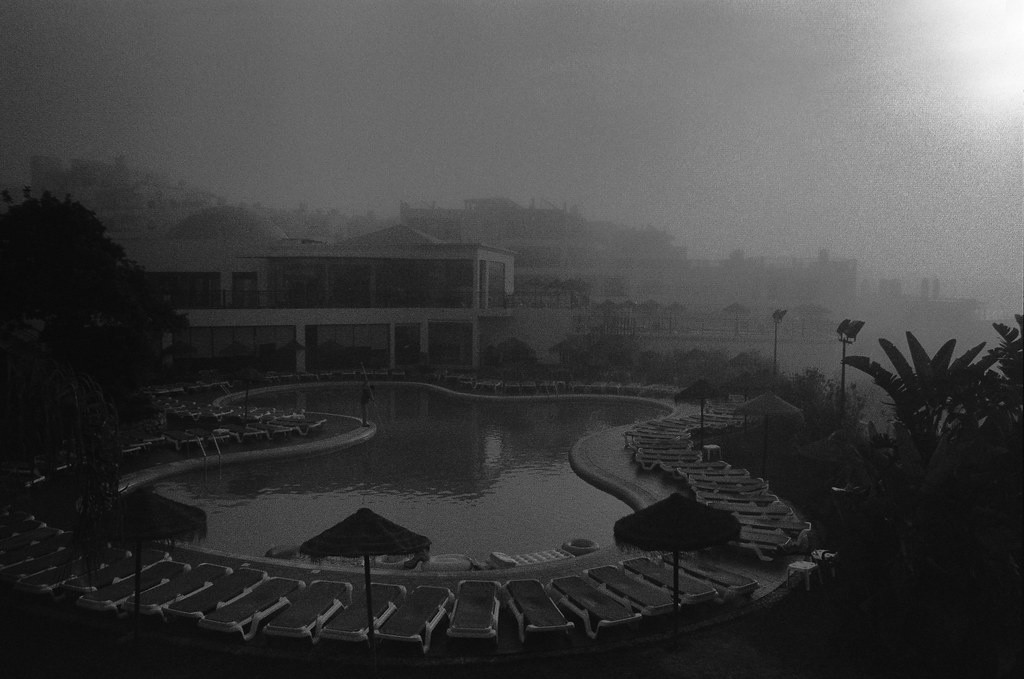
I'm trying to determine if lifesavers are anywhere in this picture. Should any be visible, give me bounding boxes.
[374,552,415,569]
[561,538,601,557]
[265,543,302,560]
[420,552,473,572]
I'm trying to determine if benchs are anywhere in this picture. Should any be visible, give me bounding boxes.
[620,381,796,516]
[545,574,643,641]
[446,579,504,648]
[656,552,762,607]
[78,558,192,620]
[501,578,575,645]
[582,564,682,623]
[261,579,354,646]
[328,367,622,396]
[121,562,235,622]
[161,567,269,623]
[374,584,456,655]
[197,576,307,641]
[0,438,170,606]
[729,511,813,547]
[320,582,407,649]
[117,368,328,460]
[618,556,719,615]
[727,526,792,563]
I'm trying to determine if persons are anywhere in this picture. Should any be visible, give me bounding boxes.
[360,379,376,426]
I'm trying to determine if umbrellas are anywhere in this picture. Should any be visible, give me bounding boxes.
[299,507,432,676]
[613,493,743,629]
[674,378,729,450]
[89,487,208,644]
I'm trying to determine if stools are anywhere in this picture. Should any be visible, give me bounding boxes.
[785,560,825,592]
